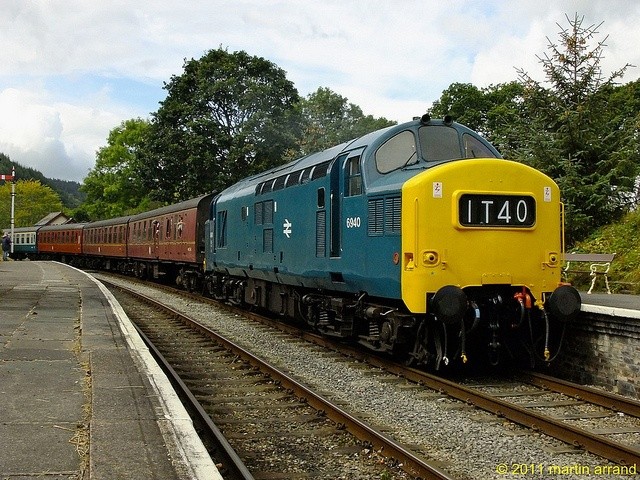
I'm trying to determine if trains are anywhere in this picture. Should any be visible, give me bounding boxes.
[0,114,582,381]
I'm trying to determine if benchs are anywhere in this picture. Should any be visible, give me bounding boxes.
[561,254,616,294]
